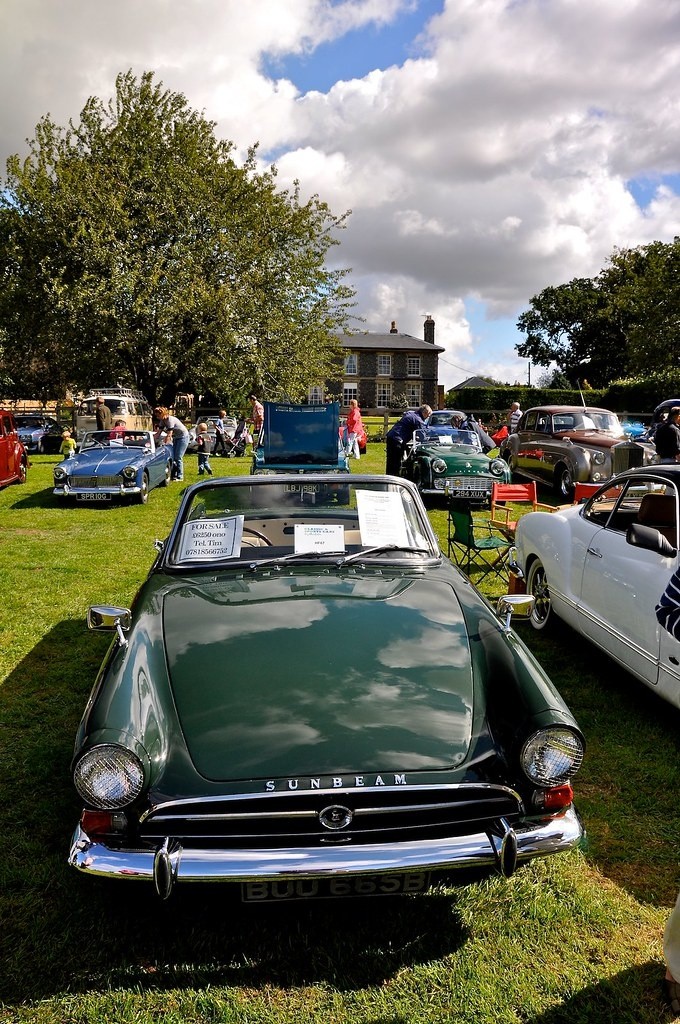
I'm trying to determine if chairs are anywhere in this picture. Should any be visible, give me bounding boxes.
[637,494,676,547]
[488,480,558,566]
[448,481,516,585]
[556,482,623,511]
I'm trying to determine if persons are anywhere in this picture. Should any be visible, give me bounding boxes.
[653,406,680,496]
[450,415,496,455]
[58,395,264,482]
[506,402,523,434]
[344,399,362,459]
[384,403,432,481]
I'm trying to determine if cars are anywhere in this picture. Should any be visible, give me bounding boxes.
[631,399,680,466]
[14,414,64,455]
[188,416,237,454]
[399,429,511,510]
[500,405,658,504]
[66,473,588,900]
[339,420,366,454]
[53,431,174,504]
[250,400,350,505]
[0,409,30,488]
[514,464,680,704]
[422,410,478,446]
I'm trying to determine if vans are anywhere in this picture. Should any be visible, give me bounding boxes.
[76,388,154,441]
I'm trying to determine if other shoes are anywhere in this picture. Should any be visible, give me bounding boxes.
[198,470,204,475]
[174,478,184,481]
[213,452,216,456]
[208,469,212,474]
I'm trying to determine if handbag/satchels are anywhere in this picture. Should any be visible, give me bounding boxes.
[245,434,253,444]
